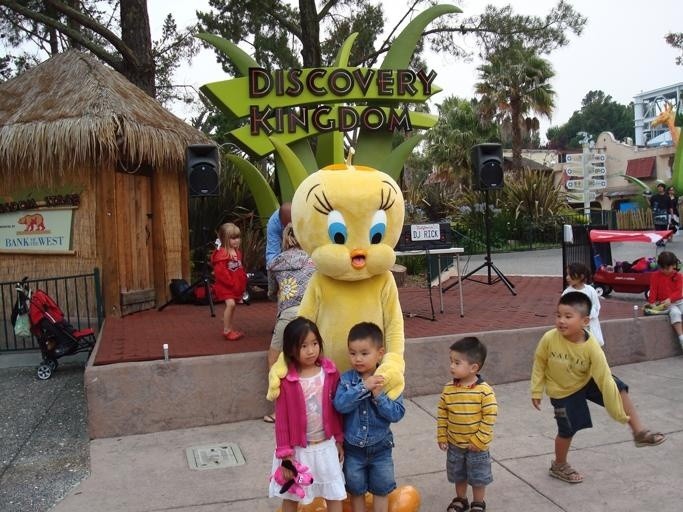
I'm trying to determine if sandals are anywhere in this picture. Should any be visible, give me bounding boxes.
[469,500,486,512]
[447,496,470,512]
[548,459,583,483]
[632,428,668,448]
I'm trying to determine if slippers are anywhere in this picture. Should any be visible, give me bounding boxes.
[263,415,276,424]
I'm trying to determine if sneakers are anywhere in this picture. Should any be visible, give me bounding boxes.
[222,329,243,340]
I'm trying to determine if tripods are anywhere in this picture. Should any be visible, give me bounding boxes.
[157,197,250,317]
[441,191,517,297]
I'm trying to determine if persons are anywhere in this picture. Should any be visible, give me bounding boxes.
[648,252,683,347]
[561,263,604,340]
[264,225,317,425]
[644,184,673,246]
[211,224,247,340]
[531,293,663,483]
[667,186,679,233]
[265,203,292,267]
[438,336,498,512]
[268,316,348,512]
[334,322,405,512]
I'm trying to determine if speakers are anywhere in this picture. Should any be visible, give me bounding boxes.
[470,142,505,192]
[184,145,222,197]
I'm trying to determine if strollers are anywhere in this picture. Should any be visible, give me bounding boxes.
[14,275,96,380]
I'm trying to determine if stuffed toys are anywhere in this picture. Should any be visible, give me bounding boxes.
[269,460,313,497]
[266,163,406,400]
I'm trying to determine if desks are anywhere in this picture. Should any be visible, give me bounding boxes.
[394,248,465,318]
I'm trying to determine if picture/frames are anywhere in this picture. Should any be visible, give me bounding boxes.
[0,183,83,256]
